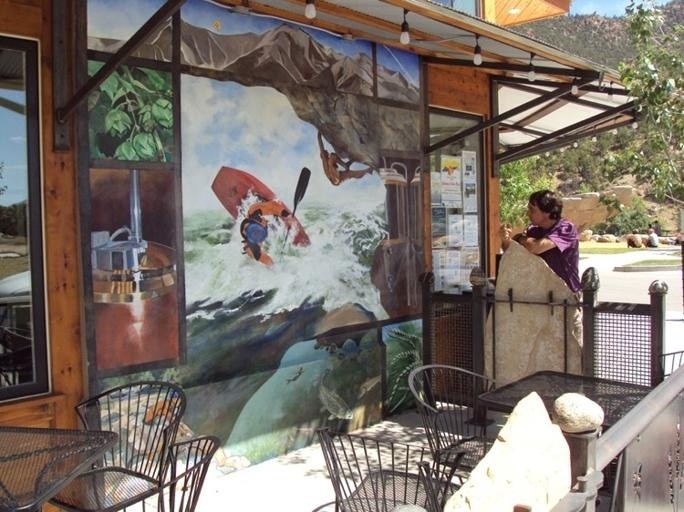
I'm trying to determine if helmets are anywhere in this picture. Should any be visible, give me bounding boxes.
[246,225,265,243]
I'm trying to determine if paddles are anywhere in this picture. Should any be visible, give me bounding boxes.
[283,167,312,245]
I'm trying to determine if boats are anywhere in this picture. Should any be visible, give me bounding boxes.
[210,165,310,247]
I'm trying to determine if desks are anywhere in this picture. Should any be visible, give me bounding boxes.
[477,370,656,432]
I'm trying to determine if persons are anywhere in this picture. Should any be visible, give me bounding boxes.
[499,184,581,309]
[234,199,298,266]
[626,220,683,248]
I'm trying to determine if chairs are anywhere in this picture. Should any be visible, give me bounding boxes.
[312,362,505,511]
[0,378,220,511]
